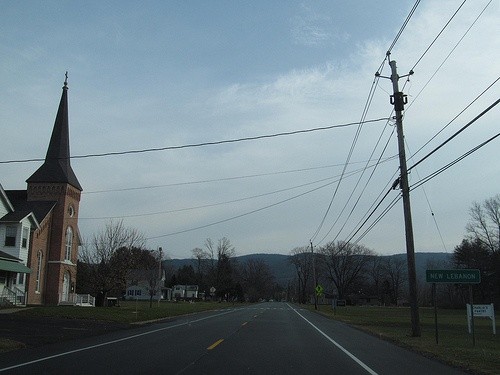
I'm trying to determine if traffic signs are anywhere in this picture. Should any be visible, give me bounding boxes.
[425,269,481,284]
